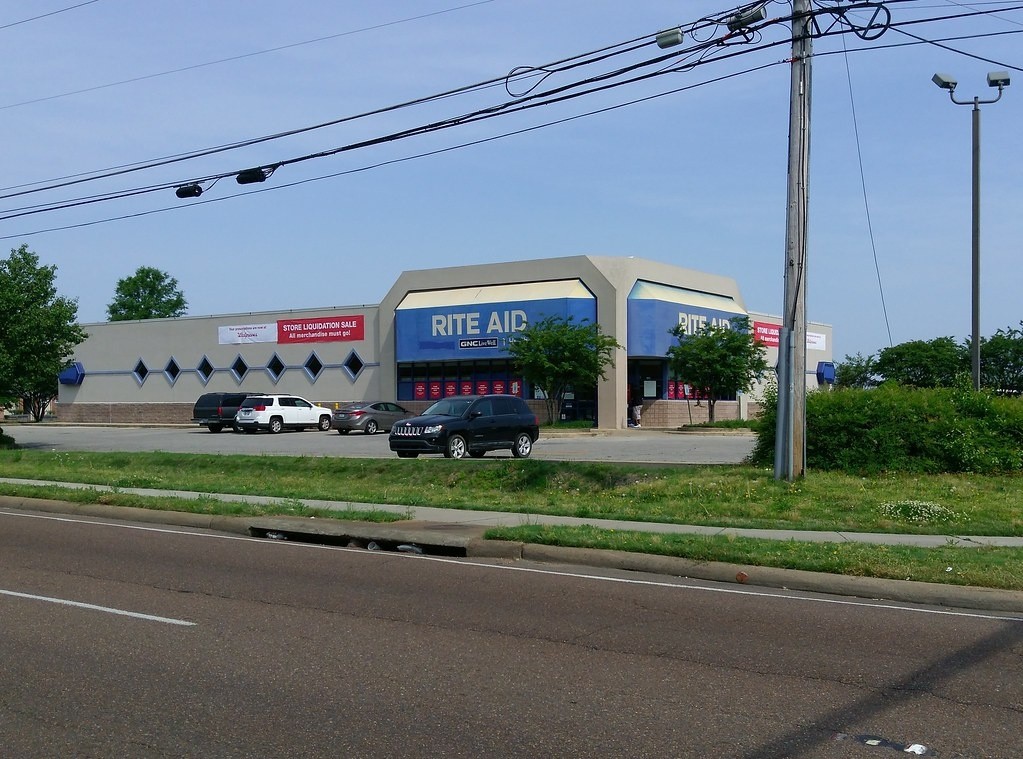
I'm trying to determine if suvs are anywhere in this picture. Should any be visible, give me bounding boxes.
[235,393,332,434]
[388,394,540,459]
[190,392,258,433]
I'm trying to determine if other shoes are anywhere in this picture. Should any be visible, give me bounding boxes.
[634,424,641,428]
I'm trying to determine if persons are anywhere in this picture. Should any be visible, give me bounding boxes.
[631,383,644,427]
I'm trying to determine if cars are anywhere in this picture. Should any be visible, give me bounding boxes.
[332,400,415,435]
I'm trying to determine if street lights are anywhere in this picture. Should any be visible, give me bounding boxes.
[931,71,1011,394]
